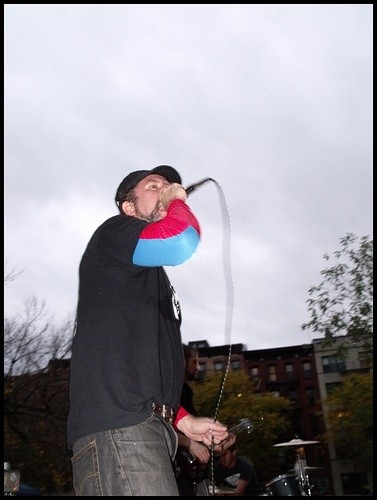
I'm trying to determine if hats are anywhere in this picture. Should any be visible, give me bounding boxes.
[117,165,182,207]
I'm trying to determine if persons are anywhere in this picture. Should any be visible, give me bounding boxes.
[66,164,228,496]
[218,444,261,496]
[175,344,199,495]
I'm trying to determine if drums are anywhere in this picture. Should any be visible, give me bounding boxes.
[264,473,307,497]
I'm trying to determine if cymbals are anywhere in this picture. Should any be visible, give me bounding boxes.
[290,466,323,472]
[273,438,321,446]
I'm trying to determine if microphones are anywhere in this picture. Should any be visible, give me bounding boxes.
[158,184,196,211]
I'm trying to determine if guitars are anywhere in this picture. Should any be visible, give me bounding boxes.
[176,415,253,482]
